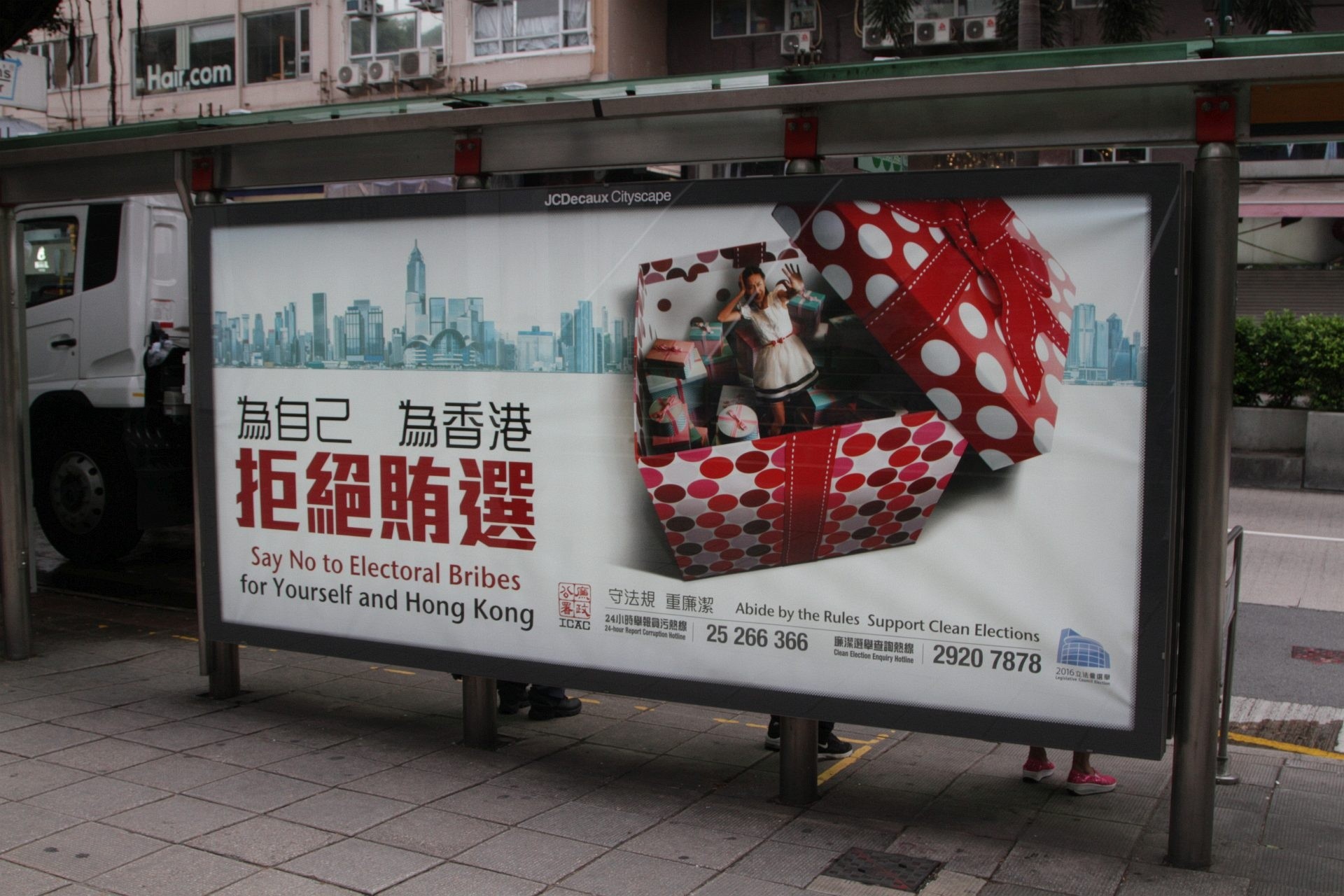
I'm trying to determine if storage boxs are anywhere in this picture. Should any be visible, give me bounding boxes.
[632,198,1076,582]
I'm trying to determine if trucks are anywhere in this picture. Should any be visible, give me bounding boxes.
[15,194,238,570]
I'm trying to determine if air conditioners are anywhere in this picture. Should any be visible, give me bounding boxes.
[914,18,949,45]
[367,59,394,83]
[337,64,363,87]
[780,31,812,55]
[964,17,1002,41]
[399,47,437,79]
[862,25,900,48]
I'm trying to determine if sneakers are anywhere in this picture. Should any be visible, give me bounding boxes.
[1066,767,1117,795]
[817,732,852,760]
[765,722,780,750]
[1022,756,1054,785]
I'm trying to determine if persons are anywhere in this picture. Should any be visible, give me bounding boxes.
[764,713,854,760]
[1022,742,1117,795]
[496,679,583,721]
[717,263,821,437]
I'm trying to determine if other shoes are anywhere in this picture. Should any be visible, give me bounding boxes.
[528,697,582,722]
[497,690,531,715]
